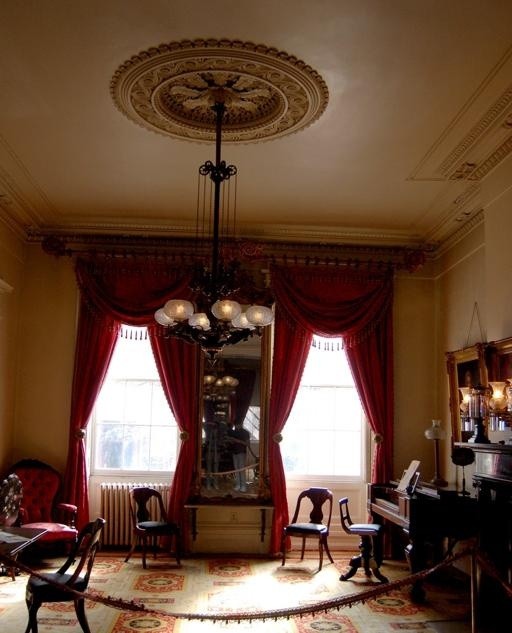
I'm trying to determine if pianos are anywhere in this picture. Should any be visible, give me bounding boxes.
[366,469,458,602]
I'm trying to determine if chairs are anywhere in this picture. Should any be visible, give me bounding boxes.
[283,486,389,587]
[123,487,182,569]
[0,457,105,633]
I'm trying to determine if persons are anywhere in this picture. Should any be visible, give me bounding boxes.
[205,420,223,489]
[225,415,250,491]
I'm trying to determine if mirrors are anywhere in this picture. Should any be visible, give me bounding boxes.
[200,325,267,503]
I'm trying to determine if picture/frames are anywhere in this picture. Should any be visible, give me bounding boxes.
[447,340,512,444]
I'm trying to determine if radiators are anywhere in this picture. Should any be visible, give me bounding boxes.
[100,483,174,550]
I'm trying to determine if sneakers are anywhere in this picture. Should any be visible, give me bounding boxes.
[233,485,247,492]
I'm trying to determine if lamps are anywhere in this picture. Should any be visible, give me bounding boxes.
[204,350,238,405]
[426,418,448,485]
[458,387,472,420]
[154,103,276,366]
[488,380,512,426]
